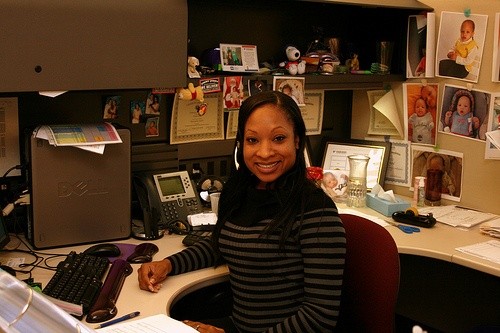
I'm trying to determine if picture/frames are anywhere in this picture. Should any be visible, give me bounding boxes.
[321,141,392,194]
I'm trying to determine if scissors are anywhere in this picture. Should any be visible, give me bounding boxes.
[384,220,420,234]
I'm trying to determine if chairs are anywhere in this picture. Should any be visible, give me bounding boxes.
[203,214,401,333]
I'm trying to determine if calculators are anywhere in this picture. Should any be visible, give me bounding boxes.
[182,224,216,247]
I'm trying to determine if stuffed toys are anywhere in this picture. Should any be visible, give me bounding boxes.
[280,46,307,76]
[188,57,199,73]
[177,84,204,103]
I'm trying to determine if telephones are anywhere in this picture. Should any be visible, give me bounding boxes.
[134,168,203,227]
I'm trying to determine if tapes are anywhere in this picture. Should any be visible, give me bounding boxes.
[405,208,419,216]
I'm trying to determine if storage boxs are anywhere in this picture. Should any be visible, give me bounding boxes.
[0,96,21,178]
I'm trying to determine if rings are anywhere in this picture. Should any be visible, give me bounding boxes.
[196,325,200,330]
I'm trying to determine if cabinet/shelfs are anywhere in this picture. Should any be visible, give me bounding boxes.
[0,0,435,93]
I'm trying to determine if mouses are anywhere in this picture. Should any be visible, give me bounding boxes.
[83,244,121,257]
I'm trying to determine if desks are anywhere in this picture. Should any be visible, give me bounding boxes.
[0,189,500,333]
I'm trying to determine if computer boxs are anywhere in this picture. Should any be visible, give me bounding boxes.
[25,123,133,250]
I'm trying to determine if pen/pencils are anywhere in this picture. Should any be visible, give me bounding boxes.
[94,311,140,329]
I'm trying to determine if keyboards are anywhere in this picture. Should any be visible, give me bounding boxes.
[42,251,110,314]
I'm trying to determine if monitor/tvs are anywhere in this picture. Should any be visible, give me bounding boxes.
[0,206,10,249]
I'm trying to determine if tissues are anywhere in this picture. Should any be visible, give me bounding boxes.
[365,182,411,217]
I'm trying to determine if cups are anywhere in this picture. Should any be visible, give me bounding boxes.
[424,169,444,205]
[210,192,221,212]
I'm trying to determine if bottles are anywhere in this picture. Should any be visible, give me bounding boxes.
[348,155,370,207]
[413,176,425,208]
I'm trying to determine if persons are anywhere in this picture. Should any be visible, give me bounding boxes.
[138,90,346,333]
[409,97,434,146]
[225,79,240,109]
[444,90,480,138]
[283,85,299,105]
[429,156,455,196]
[322,172,349,198]
[227,50,235,65]
[109,100,118,119]
[437,20,478,79]
[421,84,437,108]
[104,99,112,118]
